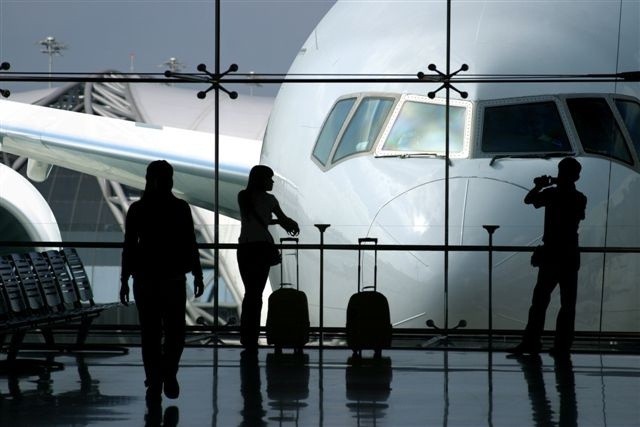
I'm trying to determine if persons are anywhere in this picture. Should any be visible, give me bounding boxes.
[236,164,300,350]
[508,157,588,355]
[120,160,205,410]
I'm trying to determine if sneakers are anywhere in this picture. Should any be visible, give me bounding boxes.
[550,347,570,361]
[162,363,179,398]
[145,388,161,406]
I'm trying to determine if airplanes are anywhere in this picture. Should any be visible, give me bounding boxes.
[0,1,640,332]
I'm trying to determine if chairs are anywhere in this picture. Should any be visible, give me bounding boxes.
[0,247,128,357]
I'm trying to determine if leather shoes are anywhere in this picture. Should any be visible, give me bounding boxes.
[506,341,543,355]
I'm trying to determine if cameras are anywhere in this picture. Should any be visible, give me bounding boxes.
[534,175,558,186]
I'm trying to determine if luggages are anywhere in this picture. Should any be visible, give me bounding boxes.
[266,238,309,354]
[346,238,392,360]
